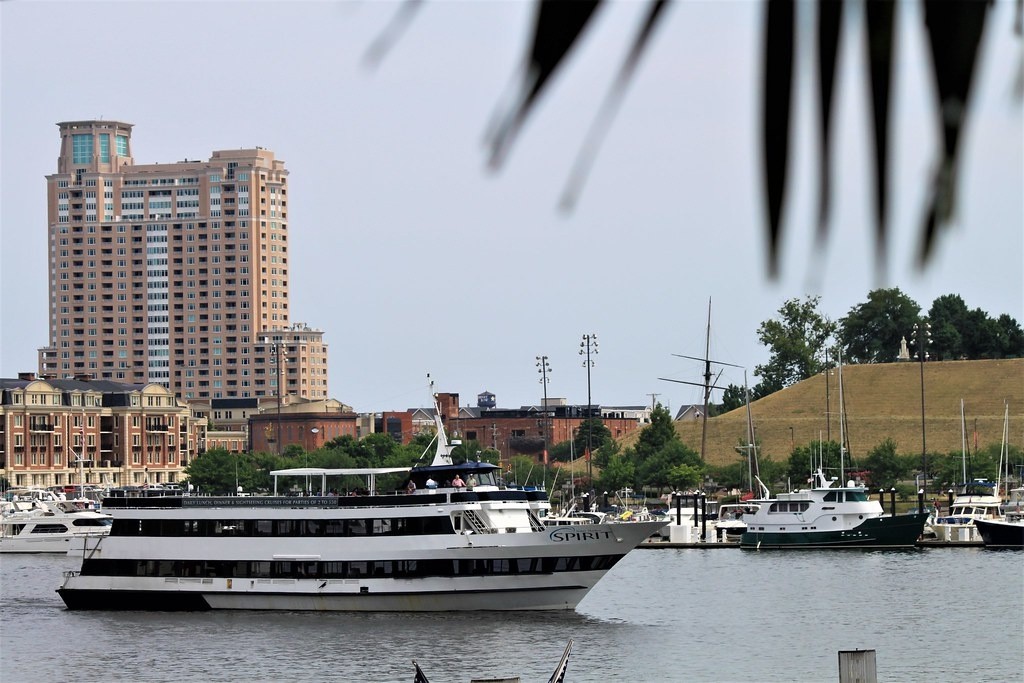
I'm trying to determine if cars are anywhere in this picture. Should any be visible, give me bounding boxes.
[22,484,183,501]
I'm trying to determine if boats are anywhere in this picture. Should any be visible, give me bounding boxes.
[58,373,674,611]
[0,498,113,554]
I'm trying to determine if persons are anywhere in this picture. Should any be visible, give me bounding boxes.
[425,475,438,489]
[303,487,370,496]
[452,474,466,487]
[466,474,476,486]
[407,480,416,493]
[932,498,941,512]
[13,494,18,500]
[84,497,89,509]
[695,411,699,418]
[734,508,744,514]
[444,480,452,487]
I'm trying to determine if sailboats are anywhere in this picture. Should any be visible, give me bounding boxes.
[740,348,1024,549]
